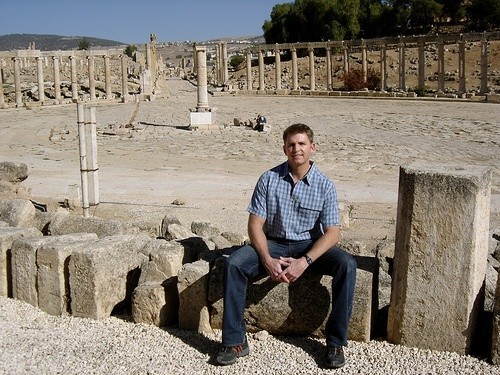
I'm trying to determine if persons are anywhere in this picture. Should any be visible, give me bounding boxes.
[214,123,357,369]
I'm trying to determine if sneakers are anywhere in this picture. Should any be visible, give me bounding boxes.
[325,343,344,368]
[215,335,249,365]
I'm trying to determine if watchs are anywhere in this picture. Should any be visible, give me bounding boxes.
[302,254,313,265]
